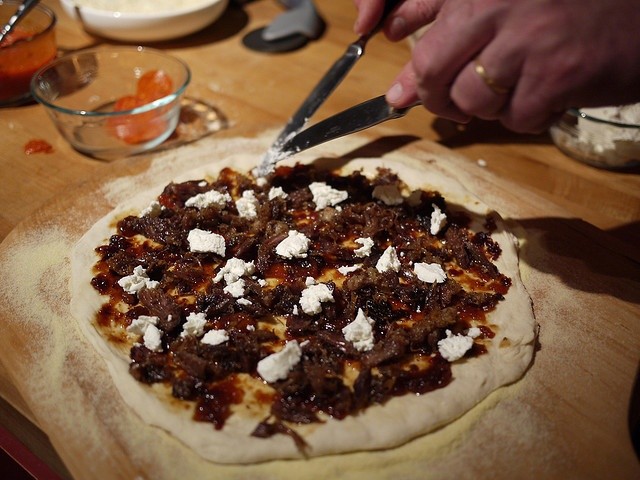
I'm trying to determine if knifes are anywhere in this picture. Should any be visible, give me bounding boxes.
[257,16,384,176]
[273,90,423,163]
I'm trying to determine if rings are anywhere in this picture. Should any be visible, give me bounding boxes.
[474,57,509,97]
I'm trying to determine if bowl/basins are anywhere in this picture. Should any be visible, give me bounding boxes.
[59,1,225,44]
[549,103,639,170]
[31,45,191,162]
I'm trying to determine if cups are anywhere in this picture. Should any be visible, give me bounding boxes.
[0,1,56,104]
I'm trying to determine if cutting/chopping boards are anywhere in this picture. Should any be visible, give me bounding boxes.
[0,1,638,479]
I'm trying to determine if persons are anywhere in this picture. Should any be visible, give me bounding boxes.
[353,0,640,135]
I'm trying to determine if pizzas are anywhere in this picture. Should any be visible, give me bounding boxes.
[70,157,539,462]
